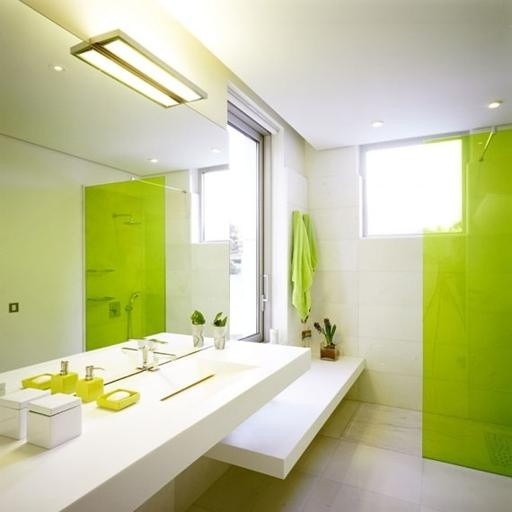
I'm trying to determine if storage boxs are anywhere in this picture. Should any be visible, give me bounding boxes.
[1,388,50,441]
[27,392,81,451]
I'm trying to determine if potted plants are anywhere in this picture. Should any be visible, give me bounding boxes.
[212,312,227,351]
[190,309,206,348]
[314,319,340,360]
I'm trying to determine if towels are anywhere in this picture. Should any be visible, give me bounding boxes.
[291,210,319,321]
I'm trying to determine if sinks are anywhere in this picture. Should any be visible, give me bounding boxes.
[143,362,241,407]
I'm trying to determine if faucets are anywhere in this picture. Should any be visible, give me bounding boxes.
[149,346,178,369]
[123,342,149,372]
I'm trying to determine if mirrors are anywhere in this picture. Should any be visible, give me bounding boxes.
[0,0,229,396]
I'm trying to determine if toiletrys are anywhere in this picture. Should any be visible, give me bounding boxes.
[51,360,79,395]
[76,365,106,402]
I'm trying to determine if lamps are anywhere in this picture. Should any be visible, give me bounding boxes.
[72,40,182,108]
[90,29,207,103]
